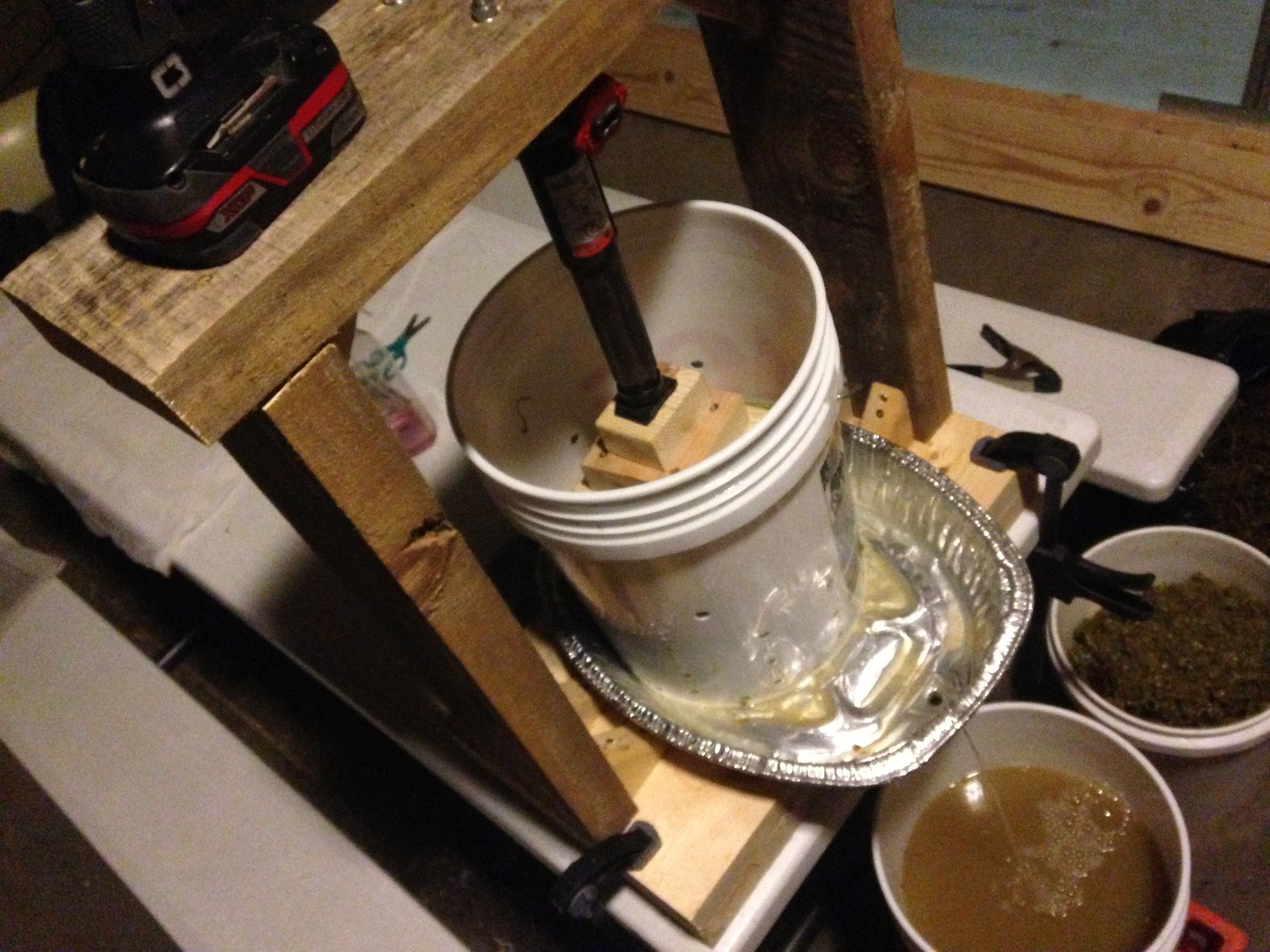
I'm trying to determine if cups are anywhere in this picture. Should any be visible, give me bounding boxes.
[348,329,437,455]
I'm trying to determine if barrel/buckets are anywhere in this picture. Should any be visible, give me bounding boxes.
[1045,526,1270,850]
[445,199,860,705]
[870,700,1191,952]
